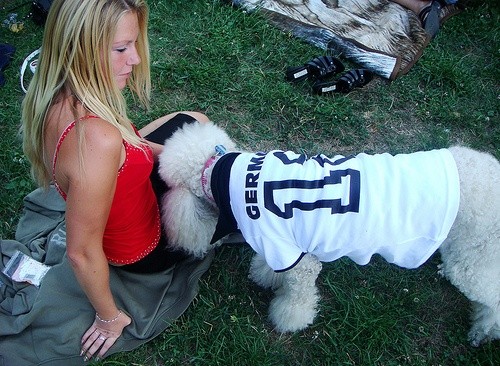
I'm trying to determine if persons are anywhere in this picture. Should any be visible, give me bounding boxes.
[393,0,441,38]
[21,0,210,363]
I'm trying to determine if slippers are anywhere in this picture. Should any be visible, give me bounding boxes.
[418,2,440,37]
[286,56,373,96]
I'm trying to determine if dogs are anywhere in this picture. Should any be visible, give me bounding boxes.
[158,120,500,348]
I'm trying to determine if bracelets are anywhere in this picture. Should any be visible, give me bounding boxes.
[96,311,122,323]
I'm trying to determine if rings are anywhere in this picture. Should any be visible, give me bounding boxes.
[98,337,106,342]
